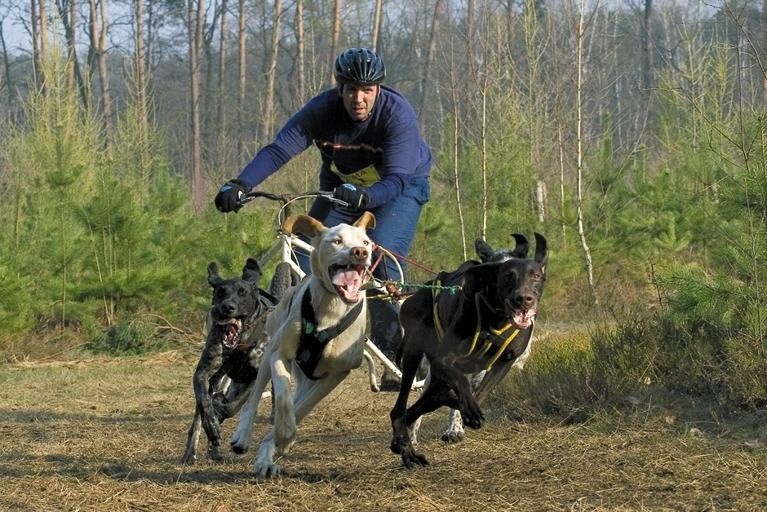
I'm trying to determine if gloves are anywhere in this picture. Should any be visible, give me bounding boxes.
[333,183,369,213]
[214,178,250,213]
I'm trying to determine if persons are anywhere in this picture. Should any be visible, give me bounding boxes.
[214,48,431,384]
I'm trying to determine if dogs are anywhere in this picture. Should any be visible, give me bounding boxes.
[180,256,279,466]
[230,210,381,479]
[389,232,550,471]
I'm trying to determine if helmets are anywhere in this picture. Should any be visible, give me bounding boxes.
[333,47,385,87]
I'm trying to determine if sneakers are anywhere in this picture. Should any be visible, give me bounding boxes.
[380,357,401,386]
